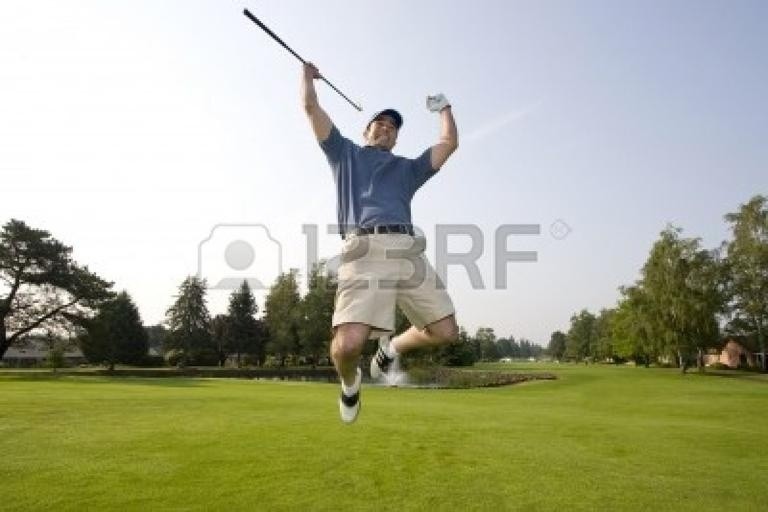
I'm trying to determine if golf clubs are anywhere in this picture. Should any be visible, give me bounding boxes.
[241,7,364,113]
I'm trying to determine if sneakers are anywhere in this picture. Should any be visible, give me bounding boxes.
[369,334,399,379]
[339,368,363,424]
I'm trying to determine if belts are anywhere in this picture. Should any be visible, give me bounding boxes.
[356,222,415,236]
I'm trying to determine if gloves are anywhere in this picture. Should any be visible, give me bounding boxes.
[425,92,451,113]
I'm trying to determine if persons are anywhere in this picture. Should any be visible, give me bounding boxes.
[301,61,458,424]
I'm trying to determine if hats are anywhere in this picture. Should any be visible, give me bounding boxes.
[366,108,402,129]
